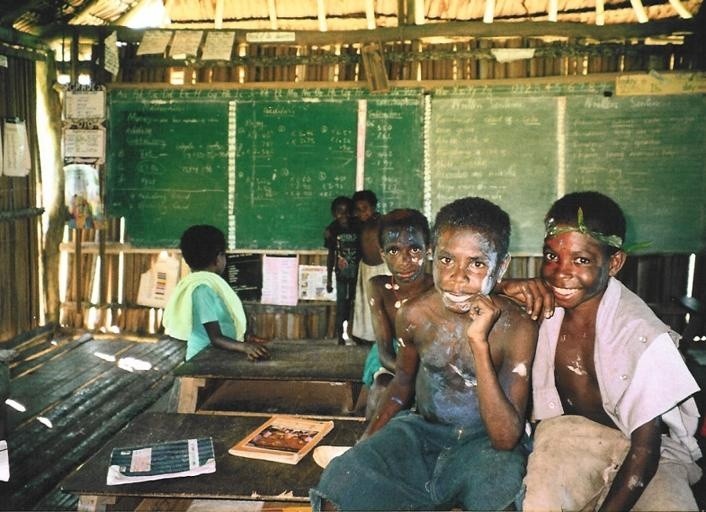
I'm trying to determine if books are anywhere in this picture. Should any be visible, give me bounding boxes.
[228,415,335,465]
[107,436,216,487]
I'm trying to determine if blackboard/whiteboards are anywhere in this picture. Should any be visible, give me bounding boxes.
[101,75,706,260]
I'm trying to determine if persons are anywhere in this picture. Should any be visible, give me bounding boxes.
[326,191,381,346]
[362,209,434,423]
[351,191,393,342]
[492,193,703,512]
[166,225,272,413]
[309,197,538,511]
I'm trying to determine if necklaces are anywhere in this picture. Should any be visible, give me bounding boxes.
[391,275,404,305]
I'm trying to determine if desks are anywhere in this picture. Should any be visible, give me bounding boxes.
[174,343,372,418]
[60,414,370,512]
